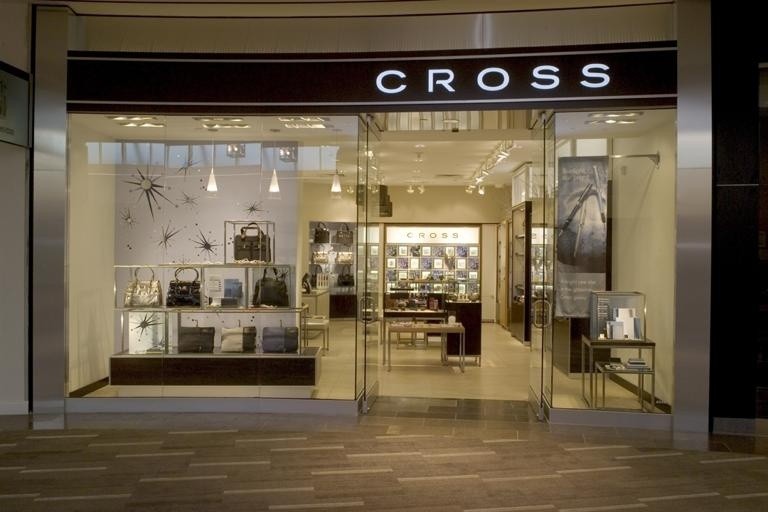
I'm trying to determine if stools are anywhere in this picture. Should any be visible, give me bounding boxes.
[298,316,330,357]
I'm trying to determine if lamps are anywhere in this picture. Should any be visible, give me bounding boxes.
[206,127,343,195]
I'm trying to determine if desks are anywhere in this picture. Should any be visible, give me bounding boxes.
[385,320,467,372]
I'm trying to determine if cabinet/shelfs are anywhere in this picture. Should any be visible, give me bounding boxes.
[113,264,308,352]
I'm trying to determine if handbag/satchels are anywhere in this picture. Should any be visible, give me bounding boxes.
[251,266,289,307]
[309,244,329,265]
[166,267,200,308]
[122,264,164,307]
[334,246,354,265]
[220,325,257,353]
[233,222,272,263]
[209,278,243,307]
[557,168,607,272]
[337,265,355,288]
[177,326,215,353]
[314,222,329,243]
[261,326,298,353]
[336,223,354,248]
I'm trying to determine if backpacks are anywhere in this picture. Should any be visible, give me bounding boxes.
[310,264,329,289]
